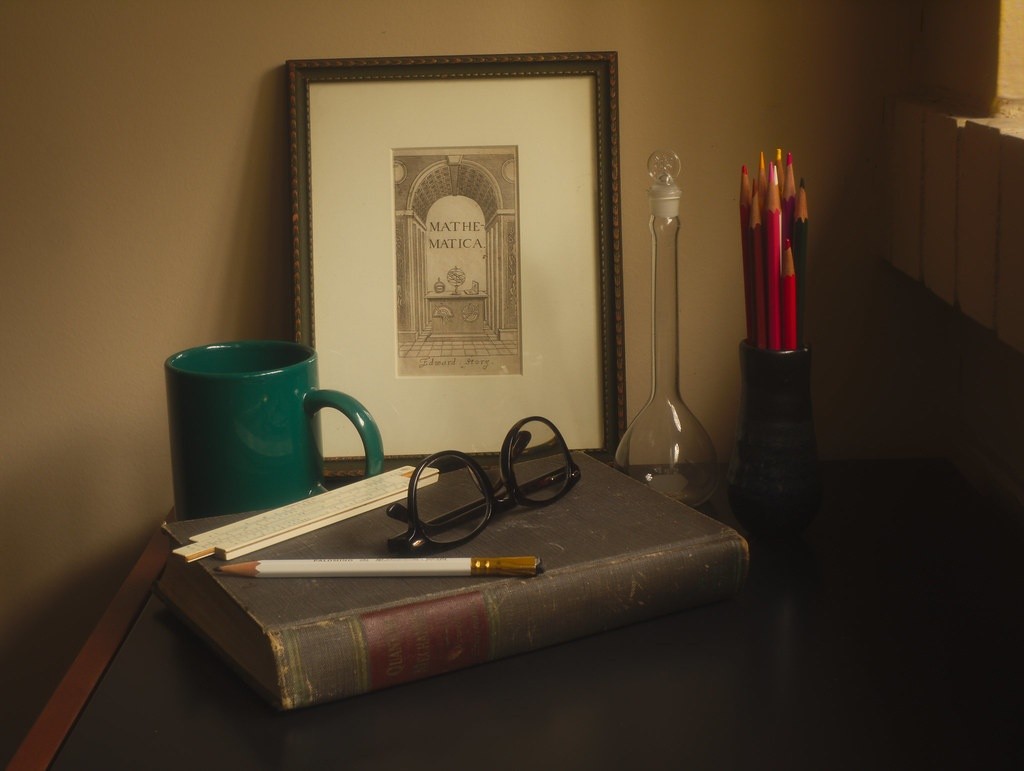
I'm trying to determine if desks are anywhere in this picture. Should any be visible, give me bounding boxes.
[0,454,1024,771]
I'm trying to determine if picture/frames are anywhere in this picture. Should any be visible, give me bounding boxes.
[286,52,629,476]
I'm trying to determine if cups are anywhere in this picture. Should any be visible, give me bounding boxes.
[163,339,384,522]
[729,335,822,546]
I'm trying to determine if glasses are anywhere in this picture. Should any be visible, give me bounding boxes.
[383,417,581,553]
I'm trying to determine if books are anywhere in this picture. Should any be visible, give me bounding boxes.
[158,452,751,711]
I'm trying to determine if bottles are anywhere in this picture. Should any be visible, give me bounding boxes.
[614,147,721,512]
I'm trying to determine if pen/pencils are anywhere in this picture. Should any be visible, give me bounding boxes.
[214,556,548,586]
[739,147,809,350]
